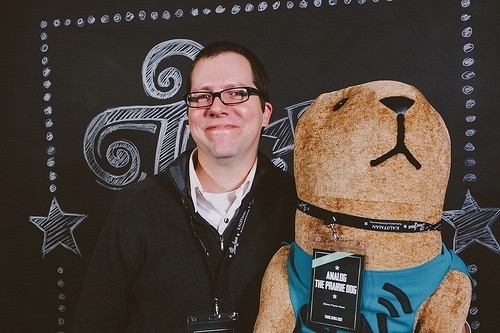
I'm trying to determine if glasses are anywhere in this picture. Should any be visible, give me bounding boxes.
[184,87,262,108]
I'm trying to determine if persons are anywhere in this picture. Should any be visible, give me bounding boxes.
[71,43,299,332]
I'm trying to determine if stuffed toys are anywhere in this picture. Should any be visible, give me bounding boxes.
[252,80,473,333]
